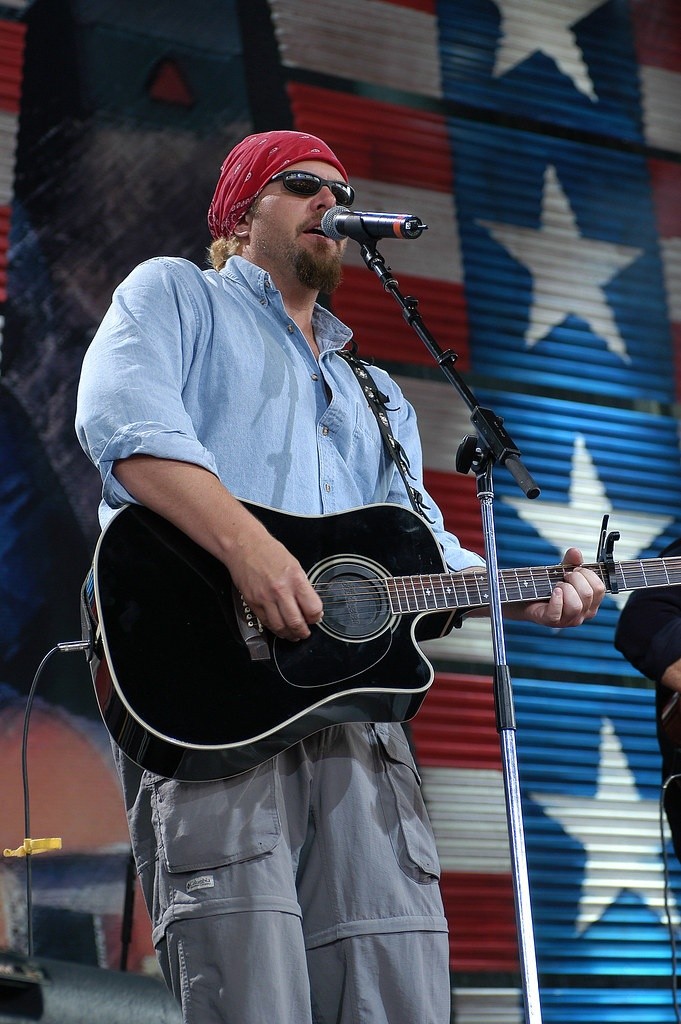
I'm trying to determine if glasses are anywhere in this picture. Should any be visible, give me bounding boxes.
[271,169,356,207]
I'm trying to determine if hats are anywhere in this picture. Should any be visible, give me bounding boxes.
[207,130,345,240]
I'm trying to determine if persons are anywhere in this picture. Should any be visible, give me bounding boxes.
[613,535,681,864]
[75,129,606,1024]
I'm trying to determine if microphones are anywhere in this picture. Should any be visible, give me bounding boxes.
[321,206,429,239]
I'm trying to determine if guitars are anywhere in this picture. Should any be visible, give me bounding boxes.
[80,487,681,790]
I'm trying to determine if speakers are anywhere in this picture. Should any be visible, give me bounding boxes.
[0,950,184,1024]
